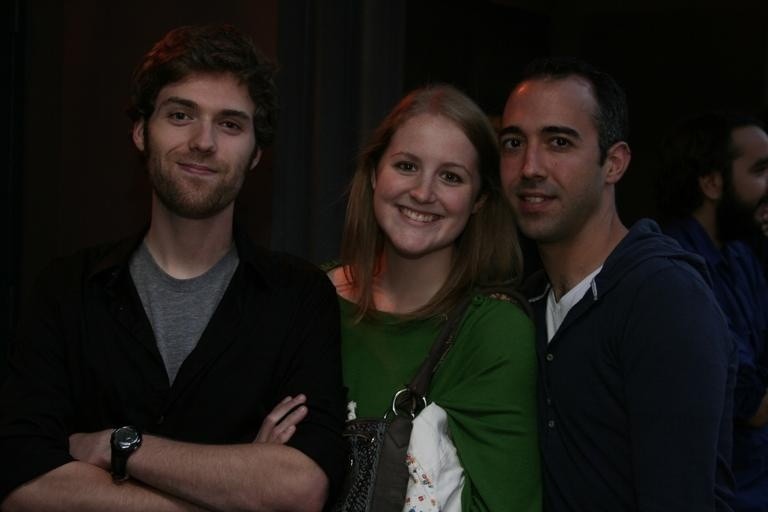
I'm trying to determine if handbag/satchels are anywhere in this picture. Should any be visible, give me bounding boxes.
[350,293,493,511]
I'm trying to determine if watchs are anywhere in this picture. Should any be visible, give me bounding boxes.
[109,425,146,481]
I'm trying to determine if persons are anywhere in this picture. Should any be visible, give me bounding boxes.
[0,24,350,512]
[318,84,543,512]
[660,120,768,512]
[494,61,740,512]
